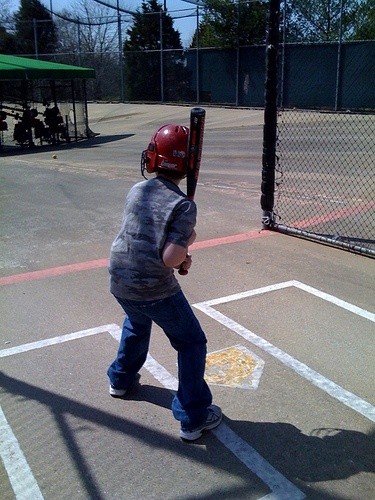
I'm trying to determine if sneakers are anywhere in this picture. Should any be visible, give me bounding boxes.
[109,384,127,396]
[179,404,223,440]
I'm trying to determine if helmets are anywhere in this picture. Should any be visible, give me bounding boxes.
[142,124,190,180]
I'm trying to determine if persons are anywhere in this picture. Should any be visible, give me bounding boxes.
[106,122,221,442]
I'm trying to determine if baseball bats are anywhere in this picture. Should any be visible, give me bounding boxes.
[178,108,206,275]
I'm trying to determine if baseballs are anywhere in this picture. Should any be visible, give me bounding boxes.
[52,154,57,159]
